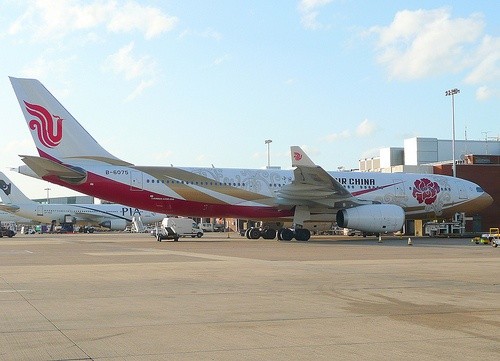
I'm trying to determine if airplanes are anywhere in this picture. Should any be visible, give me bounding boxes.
[0,171,166,233]
[5,74,493,242]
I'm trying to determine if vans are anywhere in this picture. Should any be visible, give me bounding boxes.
[161,216,203,238]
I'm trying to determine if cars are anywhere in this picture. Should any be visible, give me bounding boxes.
[490,236,500,247]
[0,226,15,238]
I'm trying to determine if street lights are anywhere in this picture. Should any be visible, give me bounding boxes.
[445,87,461,177]
[264,139,272,168]
[44,187,50,203]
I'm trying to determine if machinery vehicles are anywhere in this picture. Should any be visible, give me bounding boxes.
[471,227,500,244]
[152,227,180,242]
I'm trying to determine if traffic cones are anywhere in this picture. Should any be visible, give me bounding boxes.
[406,237,413,244]
